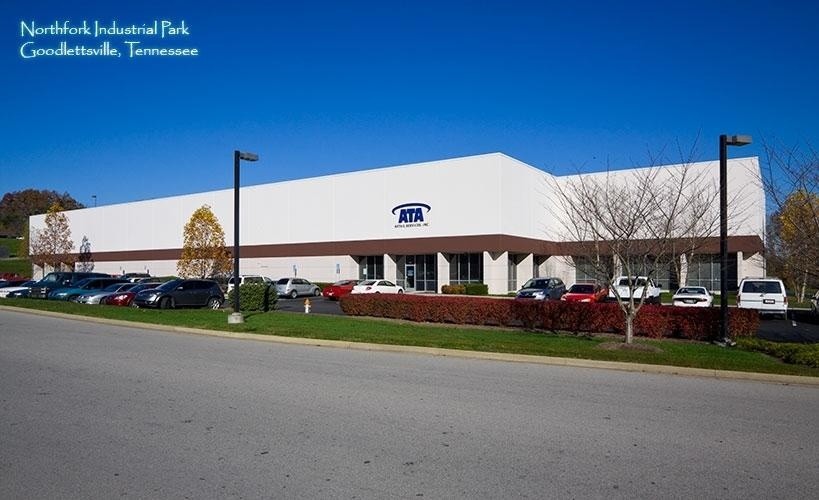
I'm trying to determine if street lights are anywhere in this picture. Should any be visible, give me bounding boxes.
[715,134,751,349]
[228,150,258,324]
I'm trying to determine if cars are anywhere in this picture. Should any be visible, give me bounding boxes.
[606,275,663,305]
[275,277,321,299]
[735,277,790,320]
[0,272,225,311]
[516,277,566,301]
[671,286,715,309]
[351,278,405,295]
[810,291,819,319]
[560,281,608,305]
[322,279,362,301]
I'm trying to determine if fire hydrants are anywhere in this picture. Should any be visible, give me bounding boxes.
[303,299,311,314]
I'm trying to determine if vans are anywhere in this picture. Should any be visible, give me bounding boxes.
[226,275,274,299]
